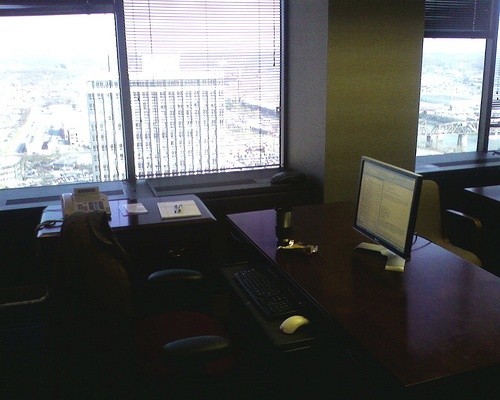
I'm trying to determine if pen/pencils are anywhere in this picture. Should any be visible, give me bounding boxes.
[174,204,182,213]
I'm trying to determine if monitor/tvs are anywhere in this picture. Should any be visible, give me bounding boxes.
[352,155,423,272]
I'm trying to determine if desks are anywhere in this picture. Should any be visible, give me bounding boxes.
[464,186,500,203]
[226,202,500,390]
[38,194,219,277]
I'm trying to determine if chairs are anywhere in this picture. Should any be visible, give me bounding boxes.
[60,210,230,400]
[415,180,483,268]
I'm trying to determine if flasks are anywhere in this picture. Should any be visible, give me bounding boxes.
[275,206,294,242]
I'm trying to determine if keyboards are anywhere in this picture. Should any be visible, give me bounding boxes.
[234,262,307,320]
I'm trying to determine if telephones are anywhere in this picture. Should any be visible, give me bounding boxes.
[60,186,115,222]
